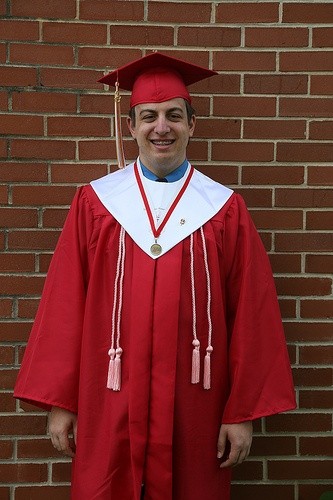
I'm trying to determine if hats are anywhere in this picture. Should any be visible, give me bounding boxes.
[96,52,218,170]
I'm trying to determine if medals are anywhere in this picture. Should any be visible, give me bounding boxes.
[150,239,162,256]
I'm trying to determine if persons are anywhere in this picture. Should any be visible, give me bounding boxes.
[13,49,297,500]
